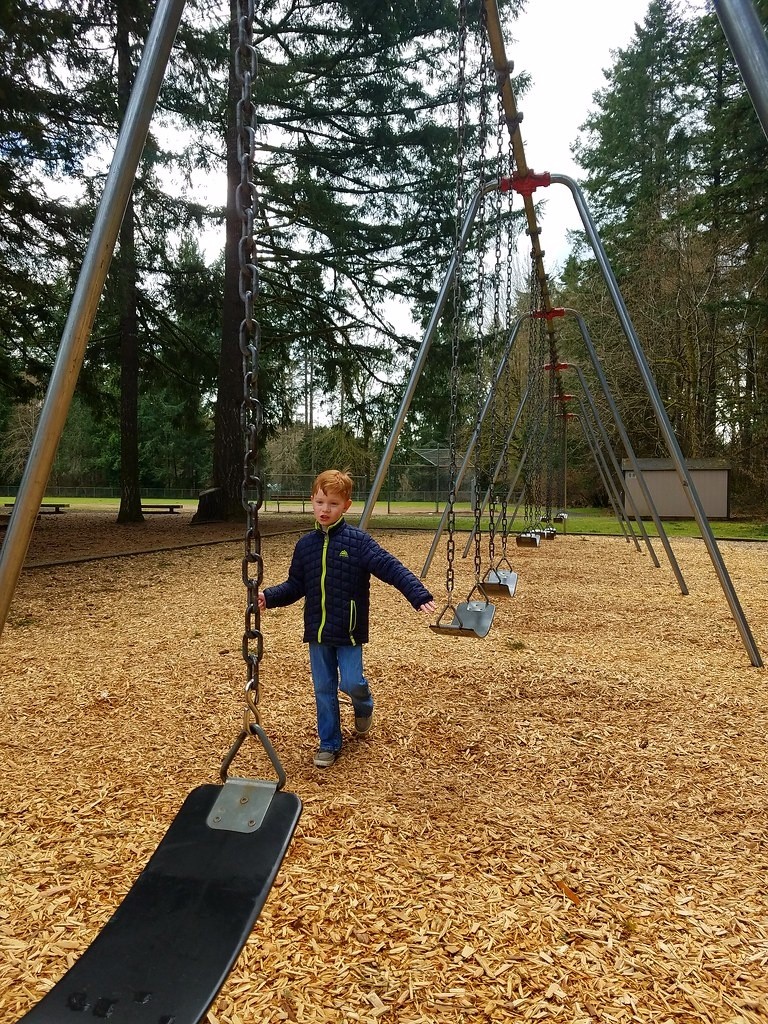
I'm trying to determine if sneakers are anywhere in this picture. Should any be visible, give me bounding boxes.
[354,713,372,734]
[313,749,342,766]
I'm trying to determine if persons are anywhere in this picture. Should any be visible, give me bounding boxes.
[259,470,438,765]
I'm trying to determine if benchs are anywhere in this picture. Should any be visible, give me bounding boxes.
[271,495,312,512]
[142,505,183,515]
[5,503,69,514]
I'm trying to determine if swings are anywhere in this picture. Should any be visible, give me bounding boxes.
[514,246,570,548]
[427,0,498,641]
[474,96,519,600]
[14,0,308,1024]
